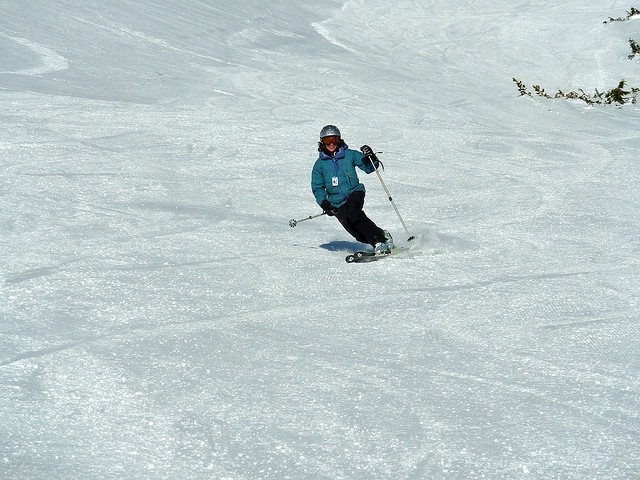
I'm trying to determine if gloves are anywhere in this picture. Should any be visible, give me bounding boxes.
[360,145,376,161]
[320,199,338,216]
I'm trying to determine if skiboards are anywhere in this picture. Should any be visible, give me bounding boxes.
[345,247,409,263]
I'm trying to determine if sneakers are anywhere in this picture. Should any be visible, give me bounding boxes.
[384,230,393,249]
[374,242,391,256]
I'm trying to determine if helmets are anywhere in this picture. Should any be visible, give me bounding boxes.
[319,125,341,139]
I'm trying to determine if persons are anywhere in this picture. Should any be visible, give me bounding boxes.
[311,125,393,256]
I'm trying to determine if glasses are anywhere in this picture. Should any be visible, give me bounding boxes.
[322,136,340,145]
